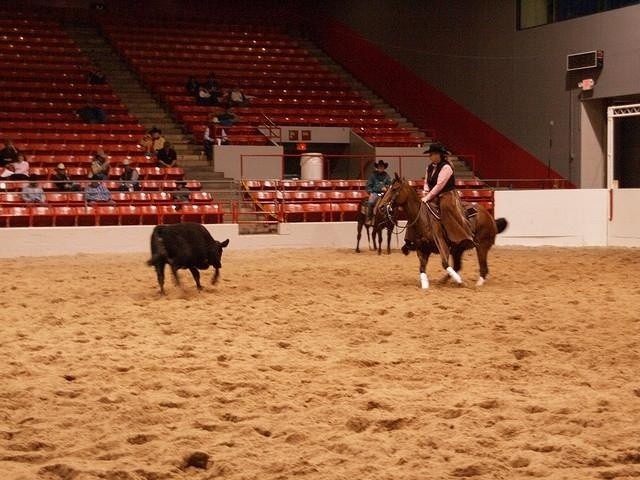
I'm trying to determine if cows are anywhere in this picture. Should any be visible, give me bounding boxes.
[146,223,229,295]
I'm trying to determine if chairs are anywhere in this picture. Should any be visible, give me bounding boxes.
[242,179,495,222]
[90,8,424,148]
[0,0,224,225]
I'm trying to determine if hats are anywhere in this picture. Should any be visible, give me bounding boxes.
[374,160,389,169]
[148,127,162,134]
[58,163,65,169]
[423,144,449,156]
[96,149,107,158]
[213,118,220,123]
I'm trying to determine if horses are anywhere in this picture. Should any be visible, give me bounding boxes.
[378,172,508,289]
[356,183,397,256]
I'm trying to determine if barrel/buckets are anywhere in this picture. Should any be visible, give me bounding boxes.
[300,153,324,181]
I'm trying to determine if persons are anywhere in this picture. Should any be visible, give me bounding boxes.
[187,71,246,160]
[0,125,177,203]
[76,101,106,125]
[420,143,473,245]
[366,160,392,227]
[90,63,106,83]
